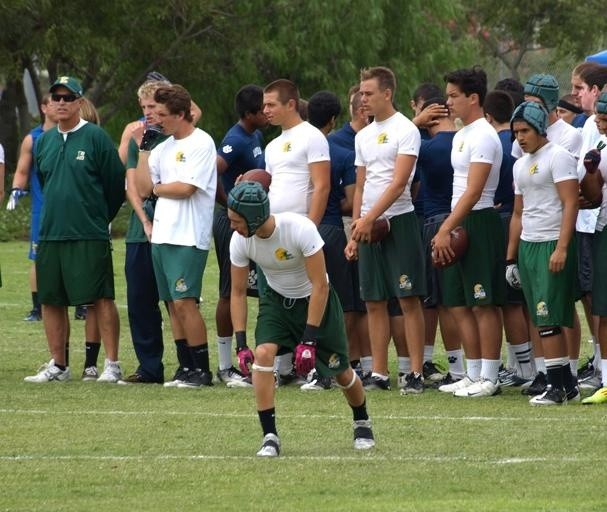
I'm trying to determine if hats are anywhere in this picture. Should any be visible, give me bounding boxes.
[49,76,83,97]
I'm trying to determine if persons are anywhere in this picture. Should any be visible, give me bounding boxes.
[215,53,607,404]
[11,76,127,383]
[115,73,226,393]
[226,180,375,460]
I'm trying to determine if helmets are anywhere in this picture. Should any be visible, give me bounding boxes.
[593,91,607,114]
[524,73,560,113]
[510,101,549,138]
[227,180,271,237]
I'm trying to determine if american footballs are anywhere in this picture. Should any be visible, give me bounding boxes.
[365,216,390,242]
[432,226,467,268]
[240,169,271,193]
[581,169,604,204]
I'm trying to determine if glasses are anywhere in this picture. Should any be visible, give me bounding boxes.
[51,93,80,102]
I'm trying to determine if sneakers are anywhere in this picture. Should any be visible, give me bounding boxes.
[352,415,376,449]
[256,432,282,458]
[23,308,41,322]
[499,354,603,395]
[431,371,467,390]
[438,375,474,393]
[75,306,87,321]
[398,371,427,395]
[116,363,165,386]
[216,363,248,384]
[296,362,392,395]
[163,365,216,390]
[96,359,123,384]
[22,359,72,385]
[225,370,279,390]
[81,363,100,382]
[529,386,568,406]
[422,360,446,381]
[564,382,580,402]
[453,376,502,399]
[580,385,607,404]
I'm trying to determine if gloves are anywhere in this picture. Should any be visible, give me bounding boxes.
[139,127,163,152]
[236,344,255,377]
[146,70,168,82]
[505,259,522,290]
[294,344,316,378]
[6,188,29,211]
